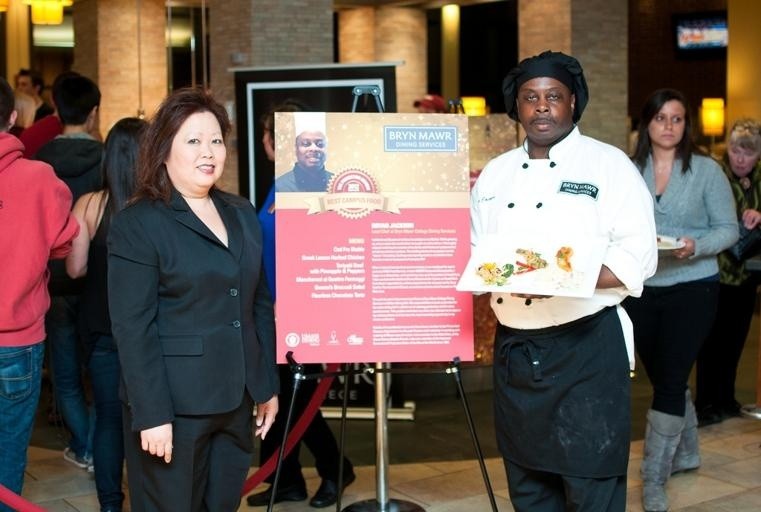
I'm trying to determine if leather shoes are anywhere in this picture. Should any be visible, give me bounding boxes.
[310,459,357,509]
[247,475,306,506]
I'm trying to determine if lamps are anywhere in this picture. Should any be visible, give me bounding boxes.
[700,98,726,143]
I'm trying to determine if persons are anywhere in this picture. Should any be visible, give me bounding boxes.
[694,117,761,423]
[248,100,354,506]
[66,117,152,511]
[37,74,108,474]
[413,90,445,112]
[465,50,658,512]
[1,78,79,512]
[623,87,740,512]
[277,132,335,192]
[0,64,82,156]
[107,88,280,512]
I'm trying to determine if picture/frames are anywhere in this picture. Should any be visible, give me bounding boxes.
[231,63,400,215]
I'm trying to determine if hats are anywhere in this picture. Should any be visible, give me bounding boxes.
[293,111,327,138]
[504,50,588,123]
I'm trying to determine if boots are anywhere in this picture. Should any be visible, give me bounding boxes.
[670,387,701,476]
[640,411,686,511]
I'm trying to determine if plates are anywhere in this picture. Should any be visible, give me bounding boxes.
[656,235,686,255]
[454,235,604,299]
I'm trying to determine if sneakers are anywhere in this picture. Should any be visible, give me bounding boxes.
[64,446,89,469]
[697,397,760,426]
